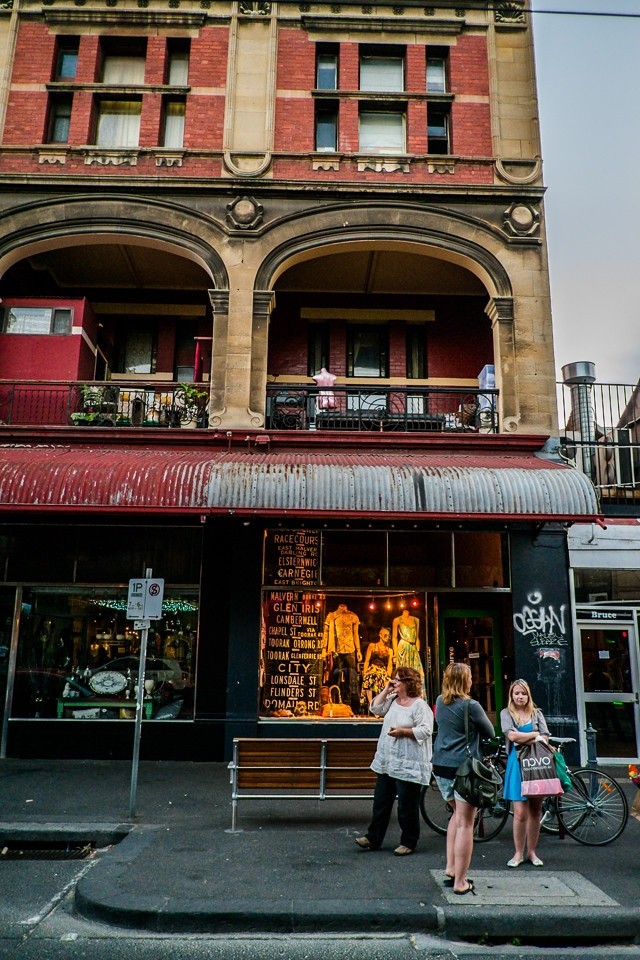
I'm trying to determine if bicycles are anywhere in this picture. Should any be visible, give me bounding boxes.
[416,732,630,846]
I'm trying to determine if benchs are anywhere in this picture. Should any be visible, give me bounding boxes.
[317,411,446,432]
[227,736,398,835]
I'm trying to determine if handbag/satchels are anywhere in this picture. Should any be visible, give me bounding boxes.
[518,734,573,798]
[452,756,503,808]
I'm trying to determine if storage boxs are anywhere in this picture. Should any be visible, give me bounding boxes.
[478,364,496,409]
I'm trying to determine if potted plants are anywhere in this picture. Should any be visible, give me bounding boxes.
[175,385,208,428]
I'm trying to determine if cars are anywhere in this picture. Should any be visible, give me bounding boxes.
[97,656,186,696]
[1,668,111,719]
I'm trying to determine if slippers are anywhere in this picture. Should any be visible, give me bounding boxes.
[443,877,473,884]
[454,884,474,895]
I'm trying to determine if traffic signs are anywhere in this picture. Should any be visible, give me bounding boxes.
[145,578,165,621]
[126,579,143,620]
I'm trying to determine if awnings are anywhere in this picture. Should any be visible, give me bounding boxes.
[0,448,601,522]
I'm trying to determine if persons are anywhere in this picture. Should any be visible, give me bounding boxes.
[586,660,627,743]
[324,604,362,715]
[391,611,426,701]
[355,667,434,855]
[32,621,64,718]
[430,663,495,895]
[360,627,393,718]
[312,368,337,409]
[500,679,551,866]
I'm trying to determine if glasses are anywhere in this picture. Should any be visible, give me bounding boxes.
[394,677,401,683]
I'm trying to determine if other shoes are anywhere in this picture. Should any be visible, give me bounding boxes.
[355,836,370,849]
[507,856,524,867]
[527,854,543,866]
[394,844,412,855]
